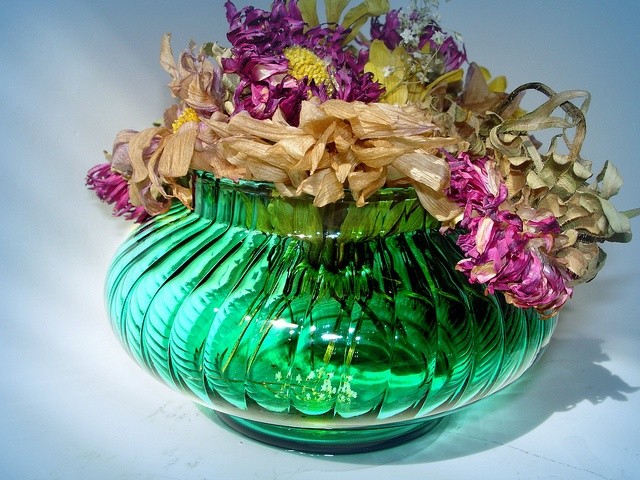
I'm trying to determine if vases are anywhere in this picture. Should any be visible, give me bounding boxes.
[100,166,563,458]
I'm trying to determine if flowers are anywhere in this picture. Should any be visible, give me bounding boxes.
[81,0,636,321]
